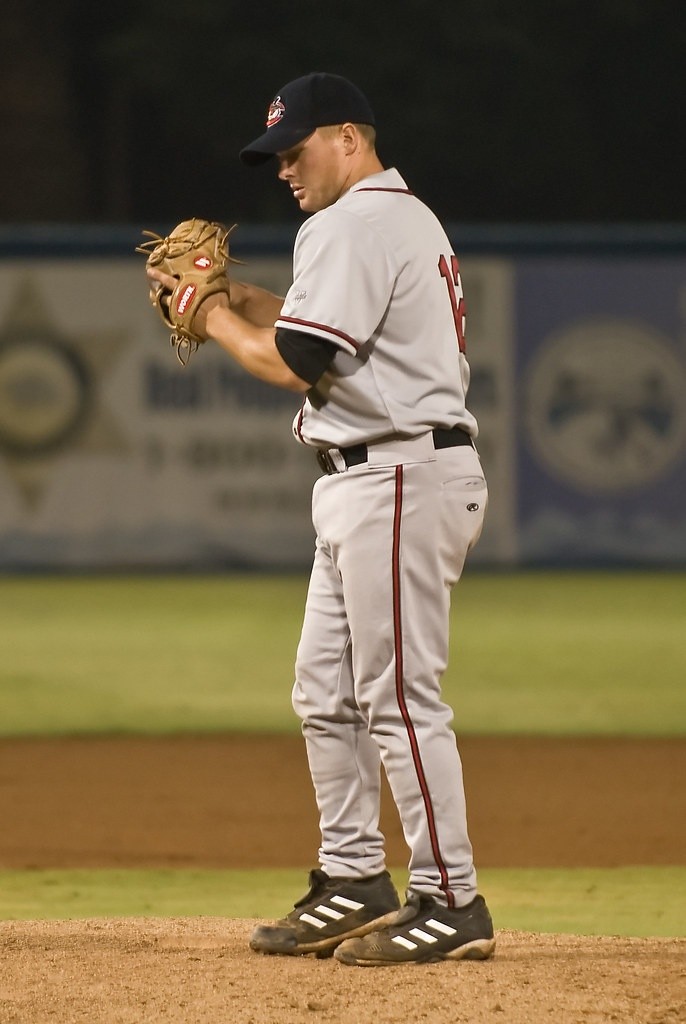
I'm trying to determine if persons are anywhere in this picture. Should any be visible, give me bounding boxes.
[135,72,497,967]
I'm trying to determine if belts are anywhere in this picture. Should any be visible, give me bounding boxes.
[317,427,472,476]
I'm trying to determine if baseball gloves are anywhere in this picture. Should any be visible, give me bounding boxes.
[134,217,249,368]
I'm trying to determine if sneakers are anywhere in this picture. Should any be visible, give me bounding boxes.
[333,887,495,966]
[251,868,401,958]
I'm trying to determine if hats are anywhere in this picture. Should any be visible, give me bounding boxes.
[238,71,375,166]
